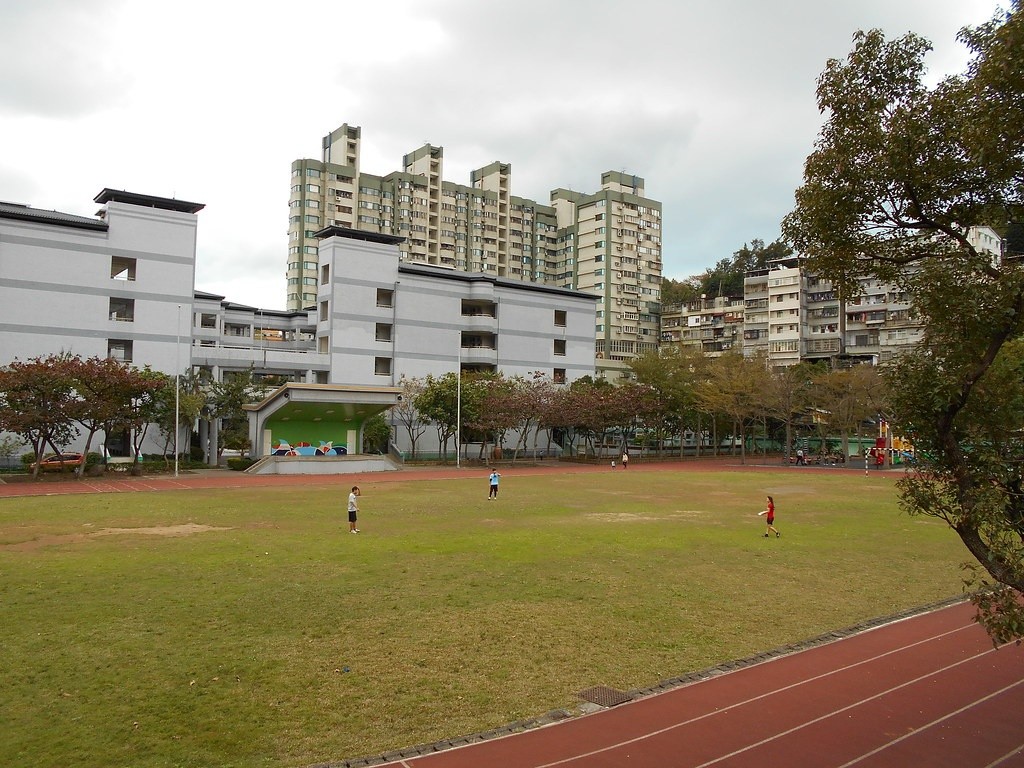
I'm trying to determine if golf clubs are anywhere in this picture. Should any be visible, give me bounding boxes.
[356,509,360,534]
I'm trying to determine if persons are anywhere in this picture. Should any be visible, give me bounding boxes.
[347,486,361,534]
[824,449,845,467]
[796,448,805,466]
[622,451,628,469]
[540,450,543,460]
[488,467,501,500]
[612,460,616,471]
[758,495,780,538]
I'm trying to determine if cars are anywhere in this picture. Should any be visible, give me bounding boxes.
[30,453,84,473]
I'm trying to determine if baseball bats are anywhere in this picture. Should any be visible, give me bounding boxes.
[758,510,769,516]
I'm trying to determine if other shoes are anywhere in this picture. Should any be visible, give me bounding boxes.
[494,497,497,500]
[349,530,357,534]
[488,497,491,500]
[355,528,361,532]
[762,533,769,537]
[776,533,781,537]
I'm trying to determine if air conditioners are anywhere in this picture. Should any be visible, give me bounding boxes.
[545,227,548,280]
[335,197,341,201]
[656,296,661,299]
[637,211,647,338]
[602,374,606,377]
[633,375,636,379]
[657,242,661,245]
[620,373,624,377]
[617,219,622,333]
[483,255,487,258]
[726,313,732,317]
[391,185,414,260]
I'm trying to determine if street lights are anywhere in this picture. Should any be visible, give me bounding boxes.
[456,329,462,469]
[174,304,182,474]
